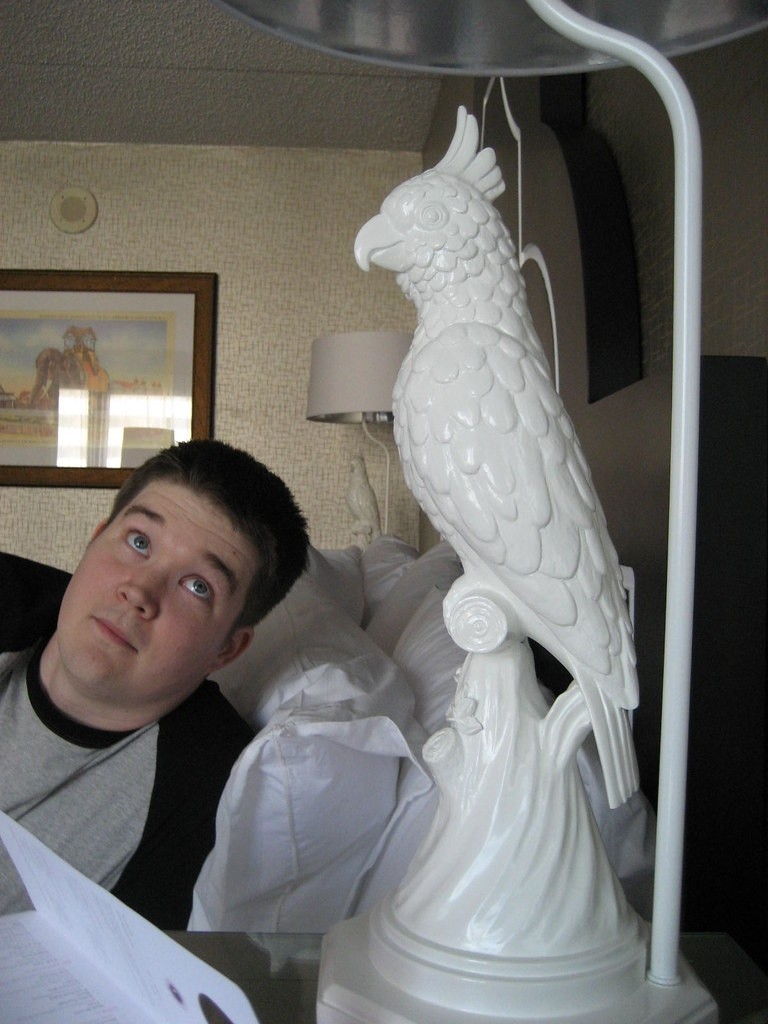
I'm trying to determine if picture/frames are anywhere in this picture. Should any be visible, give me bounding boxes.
[0,267,218,490]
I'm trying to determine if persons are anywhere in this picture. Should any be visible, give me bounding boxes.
[1,437,316,930]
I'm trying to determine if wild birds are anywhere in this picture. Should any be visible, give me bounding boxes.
[353,106,646,810]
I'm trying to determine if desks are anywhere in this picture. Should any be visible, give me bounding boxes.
[159,930,767,1024]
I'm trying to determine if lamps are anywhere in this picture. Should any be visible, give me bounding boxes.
[220,0,768,1024]
[306,331,415,535]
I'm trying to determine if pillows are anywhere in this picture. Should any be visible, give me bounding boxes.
[185,539,658,933]
